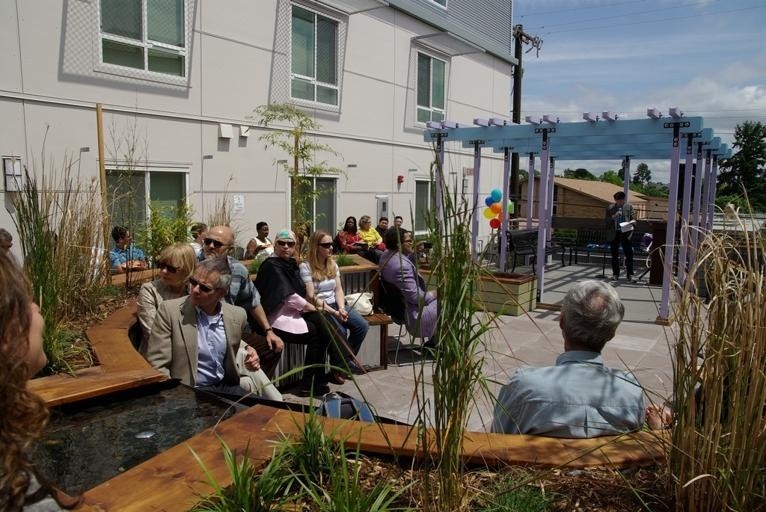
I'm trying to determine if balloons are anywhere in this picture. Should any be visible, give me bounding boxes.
[484,189,514,230]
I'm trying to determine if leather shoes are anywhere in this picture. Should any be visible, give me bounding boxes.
[327,372,346,384]
[335,361,371,375]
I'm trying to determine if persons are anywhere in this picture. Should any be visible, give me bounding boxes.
[357,216,390,264]
[489,280,645,439]
[136,244,283,402]
[380,226,444,356]
[646,371,731,430]
[248,230,331,394]
[145,256,261,403]
[604,192,637,283]
[109,226,148,274]
[389,216,407,244]
[199,225,283,391]
[375,216,388,240]
[333,216,364,254]
[0,247,65,511]
[244,221,274,259]
[298,231,369,384]
[188,222,208,262]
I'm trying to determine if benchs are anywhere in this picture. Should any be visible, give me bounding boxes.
[568,229,643,267]
[506,230,566,276]
[364,313,394,370]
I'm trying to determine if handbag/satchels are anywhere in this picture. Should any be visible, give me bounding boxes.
[344,292,374,316]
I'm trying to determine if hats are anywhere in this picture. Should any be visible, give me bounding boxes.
[274,229,297,243]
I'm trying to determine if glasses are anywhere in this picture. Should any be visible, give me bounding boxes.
[203,237,232,249]
[277,240,296,247]
[188,276,220,292]
[318,242,335,249]
[158,260,181,274]
[364,221,371,224]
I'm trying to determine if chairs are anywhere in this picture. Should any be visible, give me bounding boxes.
[378,279,408,367]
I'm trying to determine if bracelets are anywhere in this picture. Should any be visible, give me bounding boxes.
[264,327,272,333]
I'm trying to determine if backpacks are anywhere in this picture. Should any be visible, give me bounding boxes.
[315,389,374,422]
